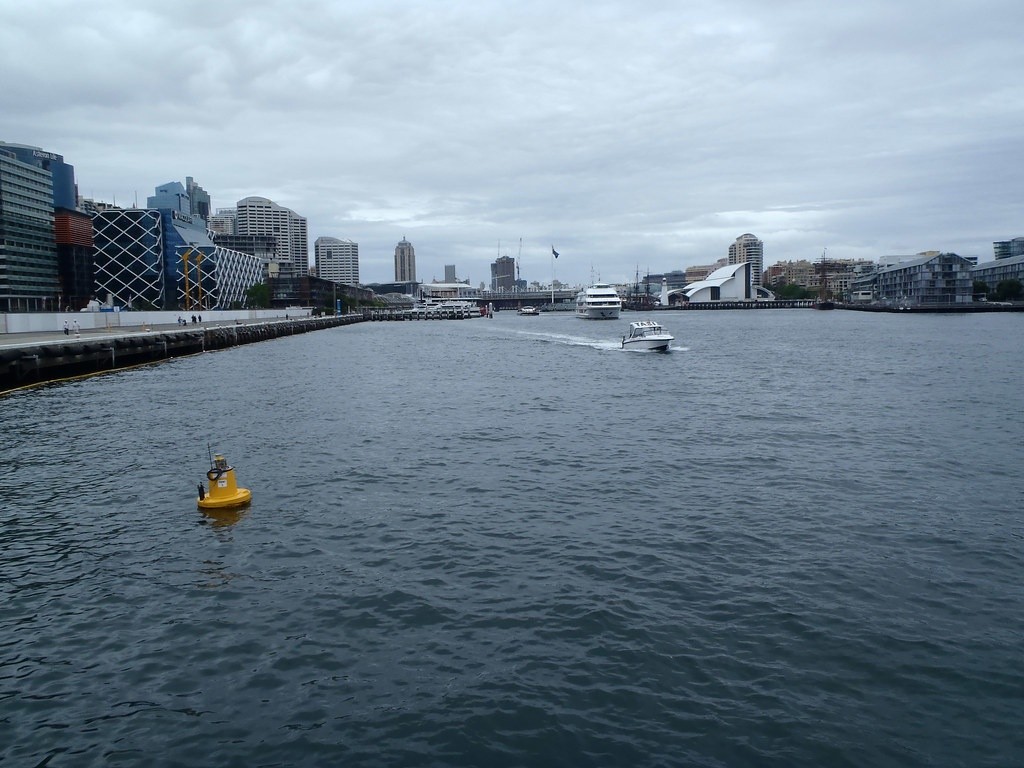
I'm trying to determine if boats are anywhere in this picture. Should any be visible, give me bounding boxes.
[516,305,540,316]
[621,321,674,353]
[576,282,623,319]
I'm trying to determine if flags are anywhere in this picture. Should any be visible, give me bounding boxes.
[553,249,559,258]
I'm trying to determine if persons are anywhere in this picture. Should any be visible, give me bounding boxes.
[286,315,288,320]
[191,314,201,323]
[72,319,80,334]
[64,320,69,335]
[178,316,186,326]
[198,482,205,500]
